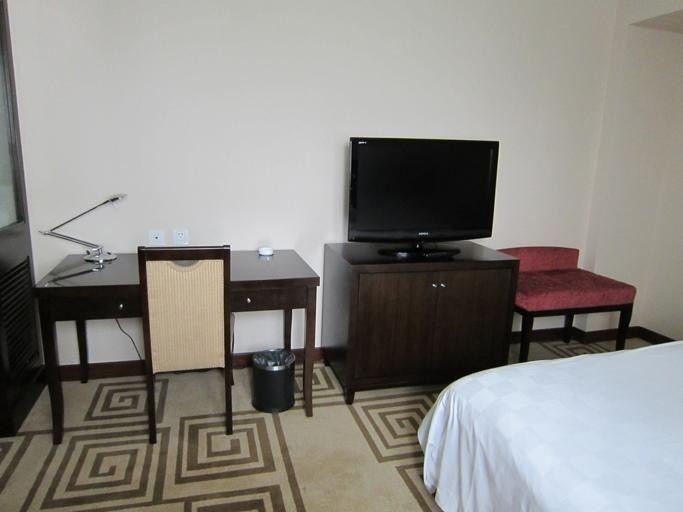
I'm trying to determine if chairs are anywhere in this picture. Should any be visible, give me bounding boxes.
[138,245,236,442]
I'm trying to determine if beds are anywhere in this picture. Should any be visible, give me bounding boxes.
[417,340,683,512]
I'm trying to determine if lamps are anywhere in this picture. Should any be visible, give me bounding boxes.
[37,195,129,263]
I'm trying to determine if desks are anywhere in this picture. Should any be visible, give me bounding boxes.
[32,249,319,444]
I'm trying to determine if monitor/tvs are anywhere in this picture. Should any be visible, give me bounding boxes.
[348,137,499,258]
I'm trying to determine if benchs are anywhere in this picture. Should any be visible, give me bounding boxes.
[495,246,636,363]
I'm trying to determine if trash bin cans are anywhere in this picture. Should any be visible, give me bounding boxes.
[252,349,295,413]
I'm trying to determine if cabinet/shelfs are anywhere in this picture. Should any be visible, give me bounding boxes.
[322,242,520,404]
